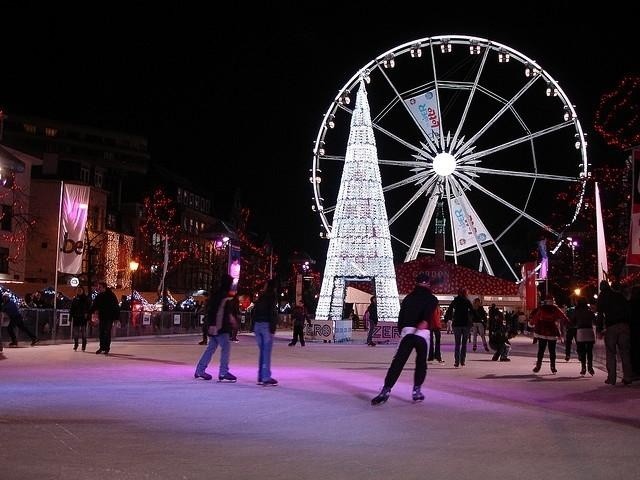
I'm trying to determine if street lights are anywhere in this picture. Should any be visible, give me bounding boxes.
[215,236,232,285]
[301,261,310,279]
[567,241,579,276]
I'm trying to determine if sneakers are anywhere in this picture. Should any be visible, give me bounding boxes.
[371,385,391,405]
[427,340,633,385]
[229,338,239,341]
[368,342,376,346]
[412,385,424,401]
[199,341,207,345]
[9,341,17,345]
[32,338,40,344]
[73,346,85,350]
[257,377,278,384]
[288,342,296,346]
[95,350,109,354]
[194,370,237,380]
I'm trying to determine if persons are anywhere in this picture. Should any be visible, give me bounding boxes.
[251,279,283,386]
[372,275,438,406]
[428,303,443,362]
[470,296,527,362]
[119,295,131,329]
[68,286,93,349]
[570,297,596,375]
[597,280,634,386]
[194,274,241,382]
[288,300,311,347]
[365,297,379,346]
[31,289,46,320]
[528,294,573,375]
[445,308,454,334]
[0,297,39,346]
[565,303,580,361]
[200,292,213,345]
[444,287,475,367]
[90,281,120,354]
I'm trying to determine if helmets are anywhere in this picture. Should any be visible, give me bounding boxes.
[458,288,468,297]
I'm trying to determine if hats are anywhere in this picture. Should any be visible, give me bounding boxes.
[415,274,430,284]
[544,296,554,302]
